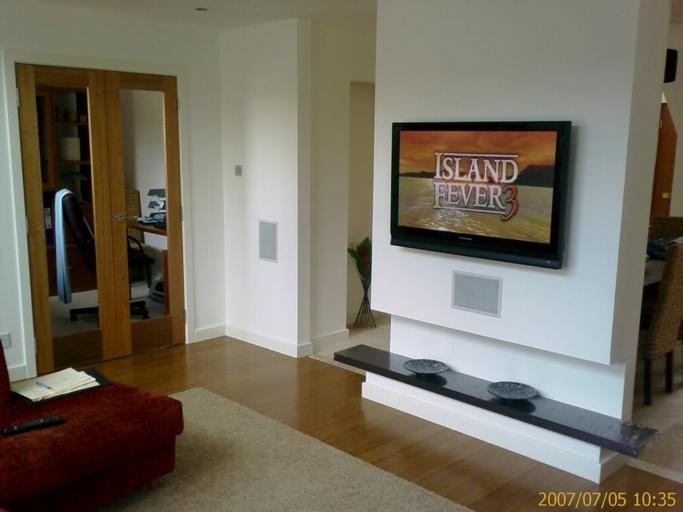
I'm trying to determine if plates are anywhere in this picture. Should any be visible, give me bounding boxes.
[402,359,449,376]
[487,380,537,403]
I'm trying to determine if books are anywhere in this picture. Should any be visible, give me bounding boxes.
[7,364,113,409]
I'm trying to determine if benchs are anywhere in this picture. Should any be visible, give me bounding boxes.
[0,340,184,511]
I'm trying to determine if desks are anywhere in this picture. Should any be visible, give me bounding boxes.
[127,218,166,236]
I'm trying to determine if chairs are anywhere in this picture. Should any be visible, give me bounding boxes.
[61,192,155,328]
[634,234,682,406]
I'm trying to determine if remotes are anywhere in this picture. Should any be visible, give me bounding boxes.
[2,416,64,437]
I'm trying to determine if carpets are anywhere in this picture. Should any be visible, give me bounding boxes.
[100,384,474,511]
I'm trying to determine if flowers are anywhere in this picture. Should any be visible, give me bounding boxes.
[346,236,372,284]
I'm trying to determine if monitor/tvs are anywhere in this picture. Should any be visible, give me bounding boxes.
[390,121,571,270]
